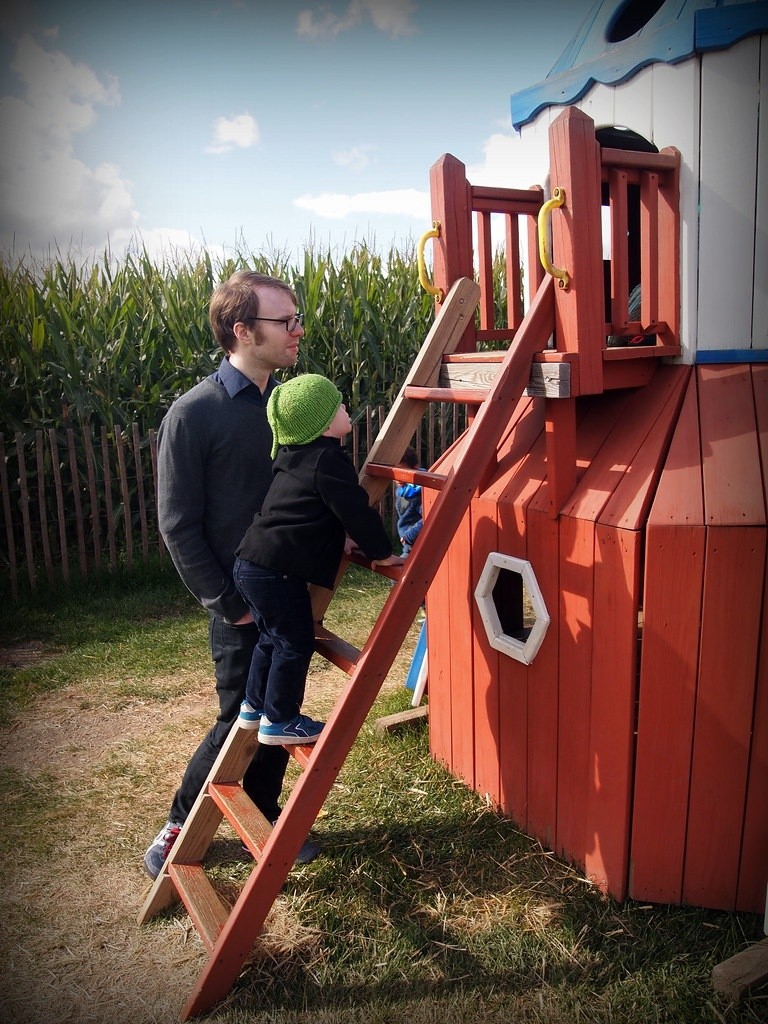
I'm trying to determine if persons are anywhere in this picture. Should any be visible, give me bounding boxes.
[234,374,404,745]
[143,271,319,880]
[395,448,427,558]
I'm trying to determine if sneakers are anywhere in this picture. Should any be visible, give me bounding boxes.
[237,699,263,730]
[143,820,183,882]
[258,712,326,745]
[242,817,317,864]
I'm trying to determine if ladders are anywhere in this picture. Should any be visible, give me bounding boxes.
[134,271,559,1024]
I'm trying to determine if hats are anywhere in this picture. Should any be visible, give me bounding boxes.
[266,374,343,460]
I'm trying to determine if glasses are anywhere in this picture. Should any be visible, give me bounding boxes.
[249,313,306,331]
